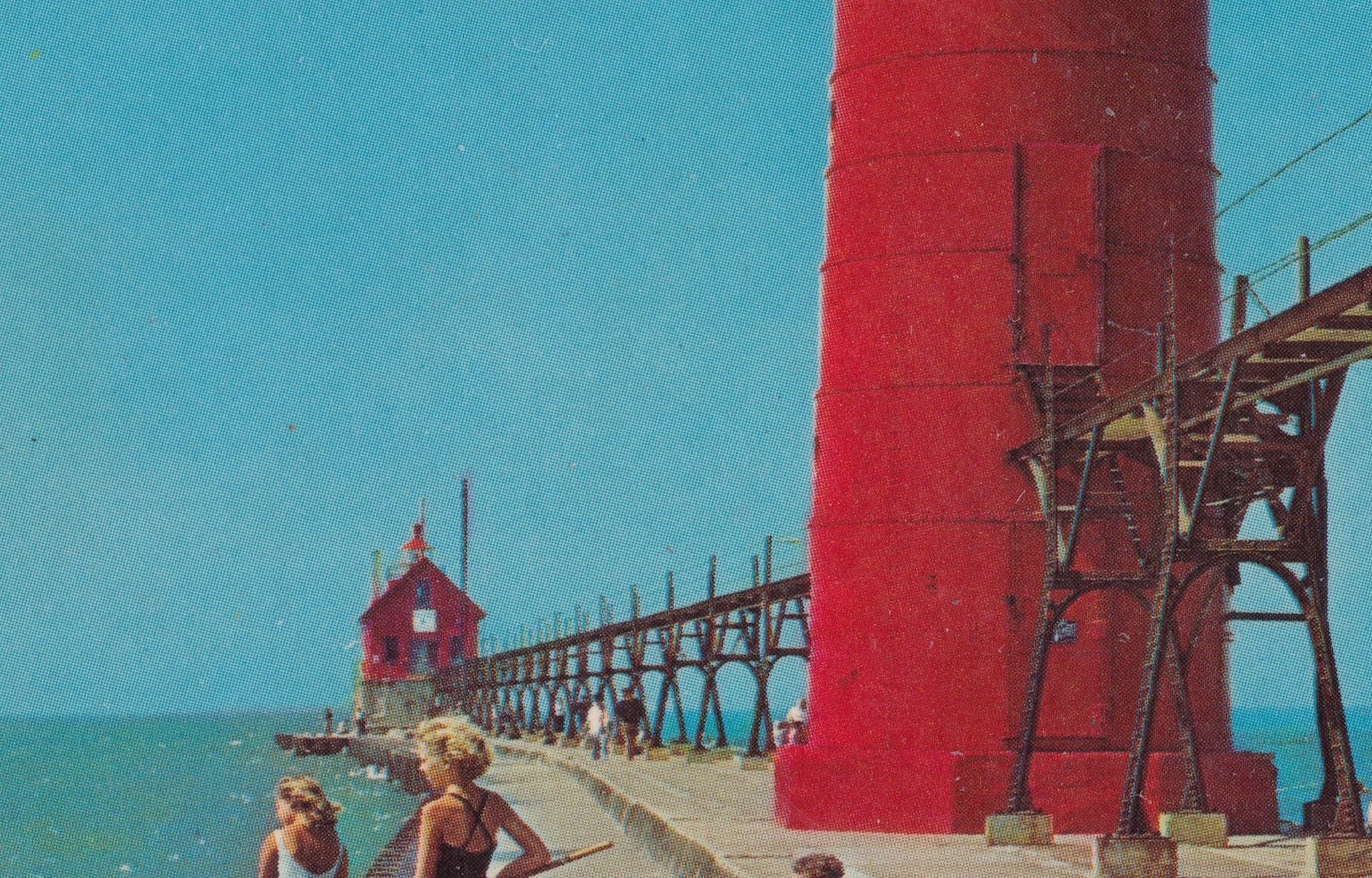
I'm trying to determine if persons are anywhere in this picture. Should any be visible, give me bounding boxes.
[354,706,367,736]
[258,775,350,878]
[553,697,567,738]
[324,706,334,738]
[586,691,610,763]
[785,697,806,745]
[616,688,650,761]
[412,716,549,878]
[790,852,844,877]
[577,694,591,731]
